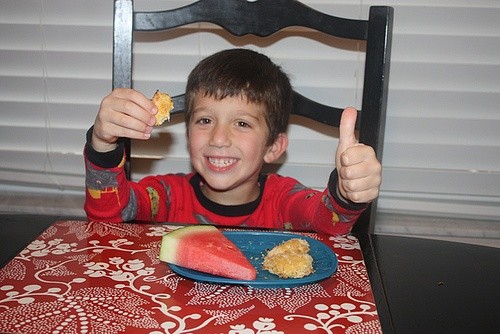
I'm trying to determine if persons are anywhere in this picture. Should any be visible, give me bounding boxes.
[83,48,382,237]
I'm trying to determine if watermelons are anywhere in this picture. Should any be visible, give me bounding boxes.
[158,225,257,281]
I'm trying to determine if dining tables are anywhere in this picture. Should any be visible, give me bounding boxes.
[1,213,500,334]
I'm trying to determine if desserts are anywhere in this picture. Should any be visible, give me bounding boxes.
[262,237,316,280]
[151,89,174,126]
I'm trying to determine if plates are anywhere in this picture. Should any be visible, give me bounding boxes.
[168,232,339,288]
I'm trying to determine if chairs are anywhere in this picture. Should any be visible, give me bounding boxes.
[114,0,393,234]
